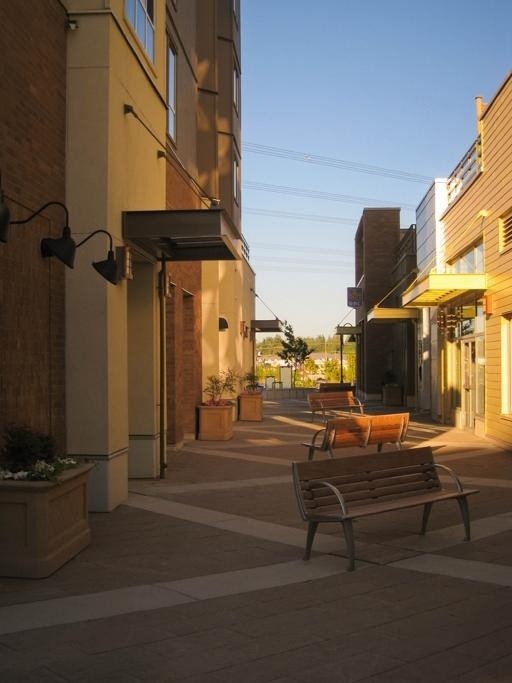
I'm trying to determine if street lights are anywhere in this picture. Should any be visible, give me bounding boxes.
[340,322,357,383]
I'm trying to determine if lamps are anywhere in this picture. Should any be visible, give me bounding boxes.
[1,201,120,286]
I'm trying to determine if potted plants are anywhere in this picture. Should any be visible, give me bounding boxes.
[380,370,402,407]
[0,422,97,580]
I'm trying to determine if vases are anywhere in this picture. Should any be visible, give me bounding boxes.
[197,394,264,441]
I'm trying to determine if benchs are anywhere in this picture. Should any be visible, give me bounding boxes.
[306,391,365,425]
[290,412,481,573]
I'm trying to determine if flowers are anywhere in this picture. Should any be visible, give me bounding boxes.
[201,367,265,407]
